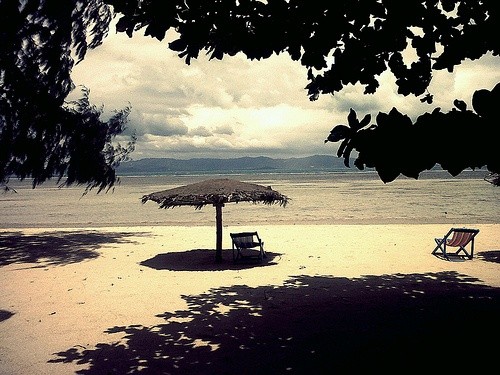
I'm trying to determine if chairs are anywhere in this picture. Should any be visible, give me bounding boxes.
[230,231,268,262]
[432,228,480,262]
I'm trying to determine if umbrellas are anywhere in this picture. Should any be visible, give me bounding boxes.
[140,178,293,265]
